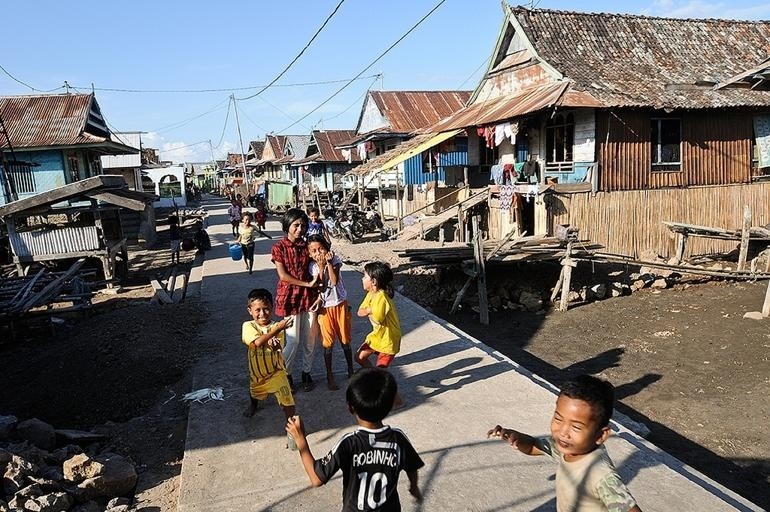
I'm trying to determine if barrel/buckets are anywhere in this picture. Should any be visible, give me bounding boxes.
[229,241,244,261]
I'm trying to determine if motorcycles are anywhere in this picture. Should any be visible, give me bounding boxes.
[320,194,384,244]
[186,182,203,204]
[245,193,266,207]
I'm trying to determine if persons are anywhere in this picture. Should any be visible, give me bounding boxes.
[306,207,332,244]
[355,262,403,413]
[192,220,212,255]
[284,368,426,512]
[241,289,295,420]
[168,215,194,266]
[270,209,335,394]
[227,194,272,275]
[484,373,643,511]
[305,235,354,393]
